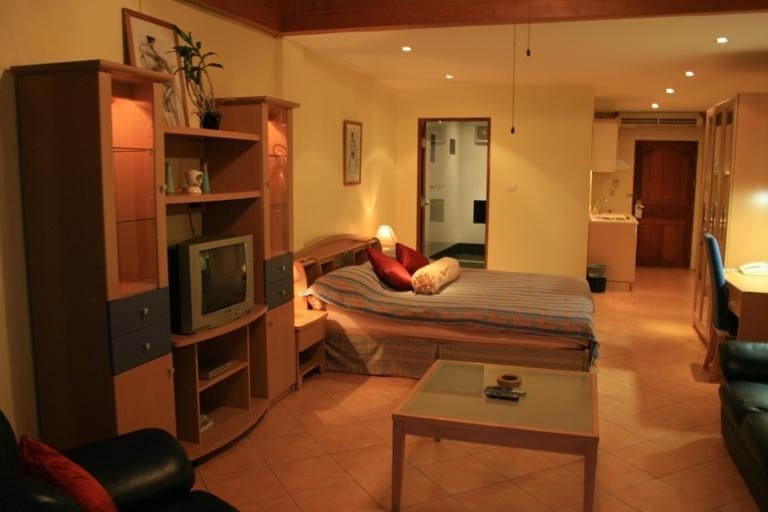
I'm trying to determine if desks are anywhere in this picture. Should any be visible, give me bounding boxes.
[392,358,600,512]
[723,267,768,340]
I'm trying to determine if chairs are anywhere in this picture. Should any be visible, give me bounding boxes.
[0,410,240,512]
[703,233,737,382]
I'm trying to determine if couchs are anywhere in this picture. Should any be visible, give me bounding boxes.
[718,340,768,512]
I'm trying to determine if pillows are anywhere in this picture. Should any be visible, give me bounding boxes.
[395,242,428,275]
[19,434,117,512]
[411,257,461,294]
[368,247,412,289]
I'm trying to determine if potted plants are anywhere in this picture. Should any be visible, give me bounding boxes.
[175,29,222,129]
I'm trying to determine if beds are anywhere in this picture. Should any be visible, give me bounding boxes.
[292,233,600,379]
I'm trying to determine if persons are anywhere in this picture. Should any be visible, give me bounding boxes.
[348,132,359,175]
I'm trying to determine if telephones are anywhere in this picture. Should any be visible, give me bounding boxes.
[739,262,768,276]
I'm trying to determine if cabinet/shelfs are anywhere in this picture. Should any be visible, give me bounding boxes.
[202,96,299,406]
[593,123,619,173]
[694,93,768,350]
[9,59,177,449]
[165,124,268,462]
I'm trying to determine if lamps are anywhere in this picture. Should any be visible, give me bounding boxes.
[293,264,300,283]
[377,224,397,250]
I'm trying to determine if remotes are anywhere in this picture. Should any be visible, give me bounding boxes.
[485,390,519,401]
[486,386,528,395]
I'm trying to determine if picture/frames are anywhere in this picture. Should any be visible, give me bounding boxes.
[122,7,189,126]
[343,120,362,185]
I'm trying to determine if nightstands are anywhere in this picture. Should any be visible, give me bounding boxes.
[295,309,329,390]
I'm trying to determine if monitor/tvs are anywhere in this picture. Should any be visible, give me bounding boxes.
[169,233,254,333]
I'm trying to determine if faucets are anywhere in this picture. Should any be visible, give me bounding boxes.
[596,196,608,207]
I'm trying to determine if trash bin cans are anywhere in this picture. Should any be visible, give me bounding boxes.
[587,264,607,292]
